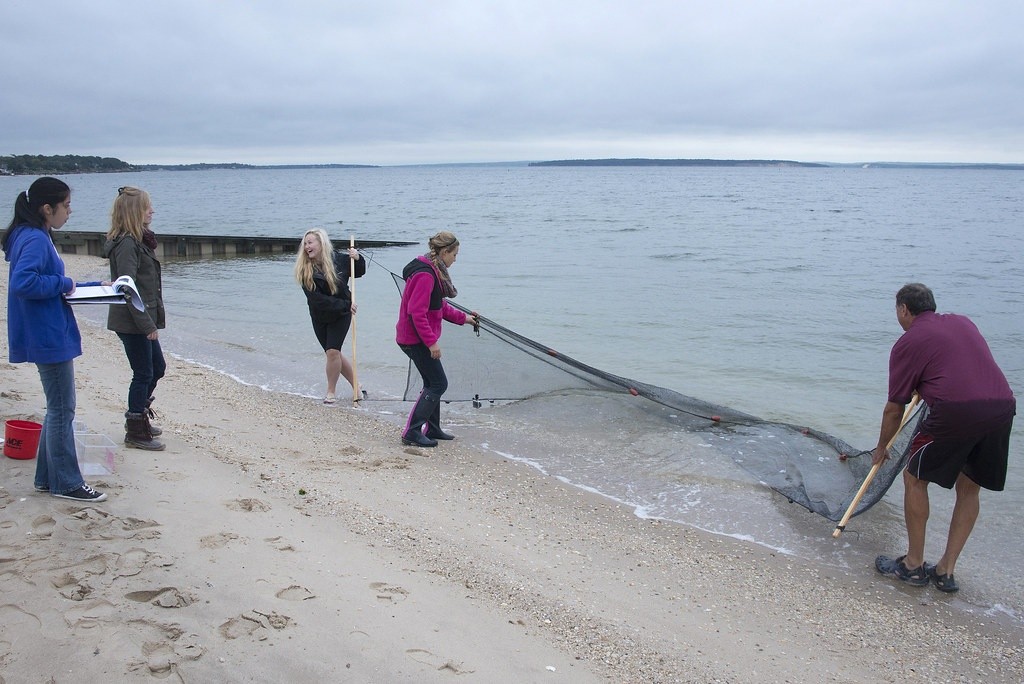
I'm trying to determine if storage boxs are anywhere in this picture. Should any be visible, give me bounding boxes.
[36,410,118,476]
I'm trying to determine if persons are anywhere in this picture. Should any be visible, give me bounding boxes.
[872,283,1017,593]
[294,228,366,408]
[4,177,115,502]
[395,230,477,447]
[105,187,167,450]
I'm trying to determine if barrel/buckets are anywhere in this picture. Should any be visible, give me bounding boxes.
[3,419,42,459]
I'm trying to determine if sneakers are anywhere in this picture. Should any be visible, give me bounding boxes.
[875,555,959,593]
[34,482,107,502]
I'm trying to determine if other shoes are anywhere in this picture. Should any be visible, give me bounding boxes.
[402,433,438,447]
[423,428,455,440]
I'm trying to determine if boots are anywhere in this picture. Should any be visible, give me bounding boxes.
[124,396,166,450]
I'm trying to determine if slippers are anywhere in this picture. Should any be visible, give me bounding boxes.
[358,390,367,401]
[324,397,336,403]
